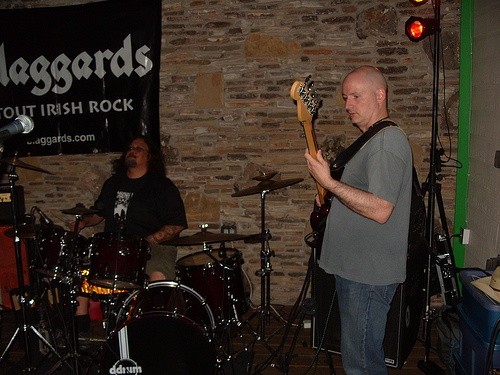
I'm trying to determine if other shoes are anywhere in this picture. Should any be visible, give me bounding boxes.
[76,314,90,334]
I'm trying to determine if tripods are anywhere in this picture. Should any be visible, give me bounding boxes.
[223,192,293,374]
[0,165,78,375]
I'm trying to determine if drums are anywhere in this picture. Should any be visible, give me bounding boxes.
[104,281,216,375]
[35,227,90,279]
[89,231,147,287]
[177,248,249,324]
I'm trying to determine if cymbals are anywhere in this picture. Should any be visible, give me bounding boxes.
[159,232,245,246]
[3,220,60,237]
[0,159,52,174]
[60,207,97,215]
[232,172,305,196]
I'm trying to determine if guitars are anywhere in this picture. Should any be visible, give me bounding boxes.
[290,74,332,238]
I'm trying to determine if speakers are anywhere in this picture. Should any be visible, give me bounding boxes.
[308,243,421,369]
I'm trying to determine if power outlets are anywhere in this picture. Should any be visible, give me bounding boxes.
[459,227,470,245]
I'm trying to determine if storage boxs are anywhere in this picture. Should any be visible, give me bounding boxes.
[450,268,500,375]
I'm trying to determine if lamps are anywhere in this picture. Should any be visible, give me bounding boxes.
[404,15,439,43]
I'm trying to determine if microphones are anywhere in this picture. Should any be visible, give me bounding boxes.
[0,114,35,142]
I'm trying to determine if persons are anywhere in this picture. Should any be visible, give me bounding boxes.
[68,137,187,333]
[305,64,413,375]
